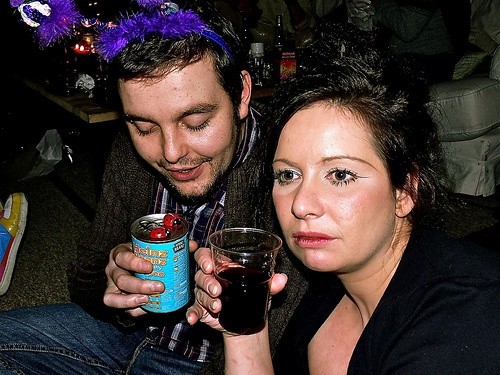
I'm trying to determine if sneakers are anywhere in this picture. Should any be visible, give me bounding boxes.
[0,192,29,296]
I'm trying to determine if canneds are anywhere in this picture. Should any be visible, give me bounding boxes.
[83,33,94,44]
[130,212,191,312]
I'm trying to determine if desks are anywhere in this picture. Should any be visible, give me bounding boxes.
[29,71,307,223]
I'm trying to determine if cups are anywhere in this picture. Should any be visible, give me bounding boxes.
[209,228,282,334]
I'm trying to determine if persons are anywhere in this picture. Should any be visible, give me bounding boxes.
[374,1,451,94]
[0,192,29,298]
[0,1,312,375]
[192,17,499,374]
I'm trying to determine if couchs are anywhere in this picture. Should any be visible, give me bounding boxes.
[362,0,500,220]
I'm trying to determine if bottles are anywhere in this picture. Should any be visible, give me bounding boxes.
[248,41,269,88]
[268,13,296,85]
